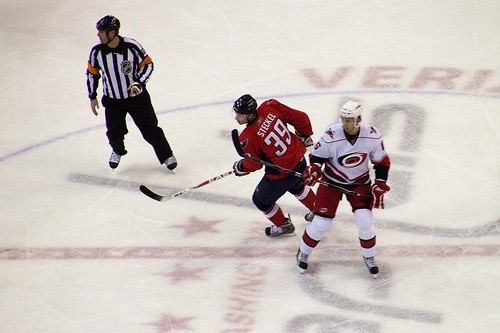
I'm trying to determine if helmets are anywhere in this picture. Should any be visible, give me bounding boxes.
[340,100,361,127]
[232,93,257,115]
[96,15,120,30]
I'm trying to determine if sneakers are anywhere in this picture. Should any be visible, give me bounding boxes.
[296,247,309,274]
[304,212,315,221]
[265,213,296,238]
[109,151,121,171]
[164,154,178,174]
[363,255,379,279]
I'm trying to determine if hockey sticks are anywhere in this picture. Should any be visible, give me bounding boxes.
[232,129,370,199]
[140,170,235,202]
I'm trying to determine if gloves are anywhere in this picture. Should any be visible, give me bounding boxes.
[302,163,321,187]
[295,130,314,147]
[371,178,390,207]
[233,159,251,177]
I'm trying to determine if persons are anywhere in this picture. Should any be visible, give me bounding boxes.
[296,100,392,279]
[84,15,178,173]
[231,93,318,238]
